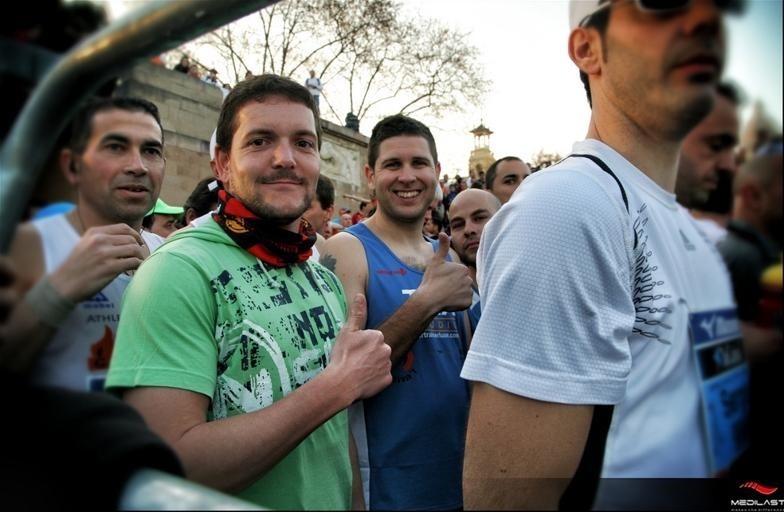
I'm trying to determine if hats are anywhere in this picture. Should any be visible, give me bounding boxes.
[144,198,185,219]
[569,1,611,29]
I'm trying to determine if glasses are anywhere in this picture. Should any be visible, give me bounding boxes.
[634,1,744,13]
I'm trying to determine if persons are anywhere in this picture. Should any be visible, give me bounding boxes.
[305,67,323,111]
[174,55,253,91]
[458,0,750,510]
